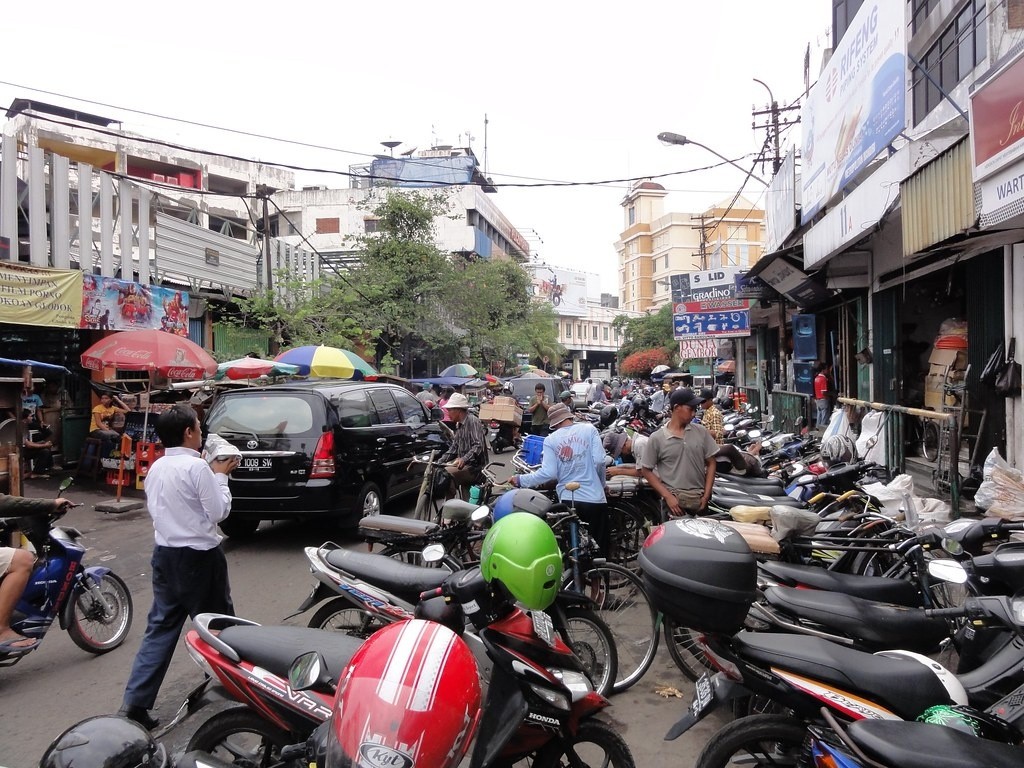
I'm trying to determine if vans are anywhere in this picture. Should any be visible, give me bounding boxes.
[199,378,458,540]
[508,377,570,432]
[693,375,713,392]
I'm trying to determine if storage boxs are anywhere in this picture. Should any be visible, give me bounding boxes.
[523,432,544,464]
[479,403,523,426]
[493,396,516,405]
[105,468,130,486]
[633,516,760,638]
[136,458,153,473]
[136,442,165,458]
[136,473,148,491]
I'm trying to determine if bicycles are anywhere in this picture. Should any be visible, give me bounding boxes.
[902,407,940,462]
[359,450,662,697]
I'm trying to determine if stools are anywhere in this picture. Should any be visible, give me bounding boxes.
[74,436,106,491]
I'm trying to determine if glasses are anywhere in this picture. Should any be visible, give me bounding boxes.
[700,399,706,404]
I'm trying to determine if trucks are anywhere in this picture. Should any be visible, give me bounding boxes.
[589,369,612,384]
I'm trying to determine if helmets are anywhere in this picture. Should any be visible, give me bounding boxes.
[559,391,574,399]
[480,512,564,610]
[38,713,179,768]
[323,618,484,768]
[492,489,554,525]
[626,392,649,410]
[504,382,514,394]
[713,397,734,409]
[600,405,617,427]
[821,435,857,467]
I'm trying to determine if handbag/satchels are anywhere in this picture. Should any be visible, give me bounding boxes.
[994,337,1022,397]
[661,488,708,513]
[979,341,1005,386]
[433,464,450,492]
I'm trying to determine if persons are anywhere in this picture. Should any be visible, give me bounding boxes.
[641,389,721,523]
[561,390,576,412]
[507,402,621,611]
[0,493,74,653]
[586,375,725,479]
[416,381,458,432]
[19,384,64,480]
[528,383,554,436]
[498,381,523,438]
[431,392,489,524]
[814,362,830,431]
[89,392,131,459]
[118,406,239,730]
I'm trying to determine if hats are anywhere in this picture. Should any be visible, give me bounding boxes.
[423,382,432,389]
[547,403,575,428]
[670,388,707,407]
[698,388,715,398]
[662,384,671,391]
[441,393,470,408]
[425,401,433,408]
[602,431,627,460]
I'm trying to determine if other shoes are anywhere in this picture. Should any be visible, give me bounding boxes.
[31,473,50,479]
[51,465,63,471]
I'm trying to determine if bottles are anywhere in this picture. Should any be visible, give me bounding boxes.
[125,422,156,442]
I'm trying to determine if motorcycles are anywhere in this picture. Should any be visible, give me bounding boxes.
[545,280,564,306]
[613,399,892,514]
[489,397,526,452]
[638,498,1024,768]
[37,714,243,767]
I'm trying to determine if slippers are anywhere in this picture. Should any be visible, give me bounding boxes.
[594,592,621,605]
[0,636,42,653]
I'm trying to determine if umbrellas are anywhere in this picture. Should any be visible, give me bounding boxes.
[439,364,478,377]
[273,344,379,381]
[203,354,301,387]
[520,364,537,371]
[79,328,219,465]
[650,365,671,374]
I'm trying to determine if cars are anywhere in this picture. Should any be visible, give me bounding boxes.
[569,382,602,408]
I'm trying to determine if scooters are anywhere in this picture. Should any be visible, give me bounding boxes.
[0,477,134,668]
[176,540,637,768]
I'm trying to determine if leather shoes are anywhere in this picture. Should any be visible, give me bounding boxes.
[117,704,160,730]
